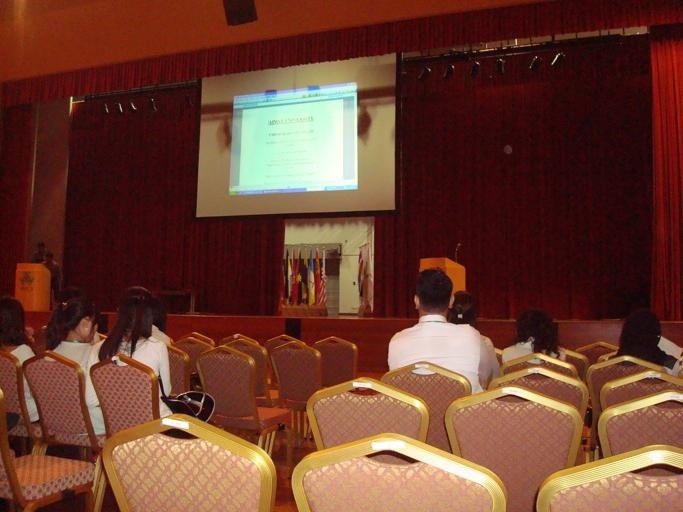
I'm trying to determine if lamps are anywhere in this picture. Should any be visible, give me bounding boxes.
[469,54,482,79]
[147,96,161,112]
[495,55,508,76]
[103,103,110,115]
[127,98,137,113]
[416,60,432,83]
[550,51,572,70]
[441,63,455,82]
[115,103,124,114]
[529,56,544,73]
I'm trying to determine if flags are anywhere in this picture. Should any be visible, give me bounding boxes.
[284,246,328,305]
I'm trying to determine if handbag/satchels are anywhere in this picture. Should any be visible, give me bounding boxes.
[157,375,217,424]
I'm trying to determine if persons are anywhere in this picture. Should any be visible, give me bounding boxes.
[387,268,485,394]
[44,253,63,296]
[447,292,503,388]
[89,287,171,422]
[608,311,679,369]
[47,297,106,444]
[504,308,566,367]
[0,299,40,432]
[32,241,49,263]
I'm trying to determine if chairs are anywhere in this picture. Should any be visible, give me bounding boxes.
[535,442,683,512]
[584,353,669,453]
[265,333,298,364]
[271,339,327,441]
[195,345,293,458]
[223,339,279,454]
[313,335,358,387]
[444,381,584,512]
[597,387,683,457]
[306,376,431,453]
[597,368,682,412]
[92,352,161,512]
[559,345,589,382]
[21,348,107,454]
[173,336,214,379]
[183,330,215,346]
[97,411,279,511]
[574,339,620,363]
[290,431,510,509]
[487,364,592,423]
[498,352,582,379]
[0,348,44,448]
[168,346,192,398]
[0,388,96,512]
[379,359,473,456]
